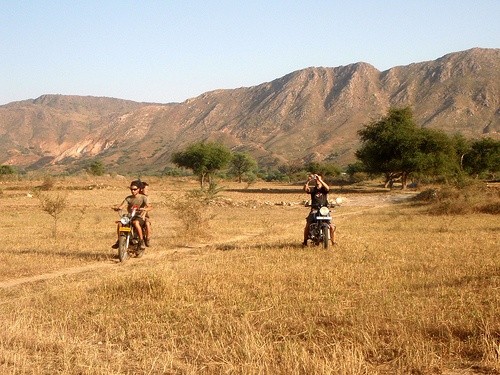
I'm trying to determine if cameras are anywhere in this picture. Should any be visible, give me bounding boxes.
[309,174,316,179]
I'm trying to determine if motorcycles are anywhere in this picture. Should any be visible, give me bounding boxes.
[112,203,145,262]
[304,203,336,249]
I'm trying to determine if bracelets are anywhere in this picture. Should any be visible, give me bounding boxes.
[308,180,310,183]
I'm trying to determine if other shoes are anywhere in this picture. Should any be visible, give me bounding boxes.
[146,238,150,247]
[138,240,146,250]
[112,242,118,249]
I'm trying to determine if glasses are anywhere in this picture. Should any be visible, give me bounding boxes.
[130,188,139,190]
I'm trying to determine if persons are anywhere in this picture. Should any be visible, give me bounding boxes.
[112,181,146,250]
[140,182,151,247]
[301,174,338,247]
